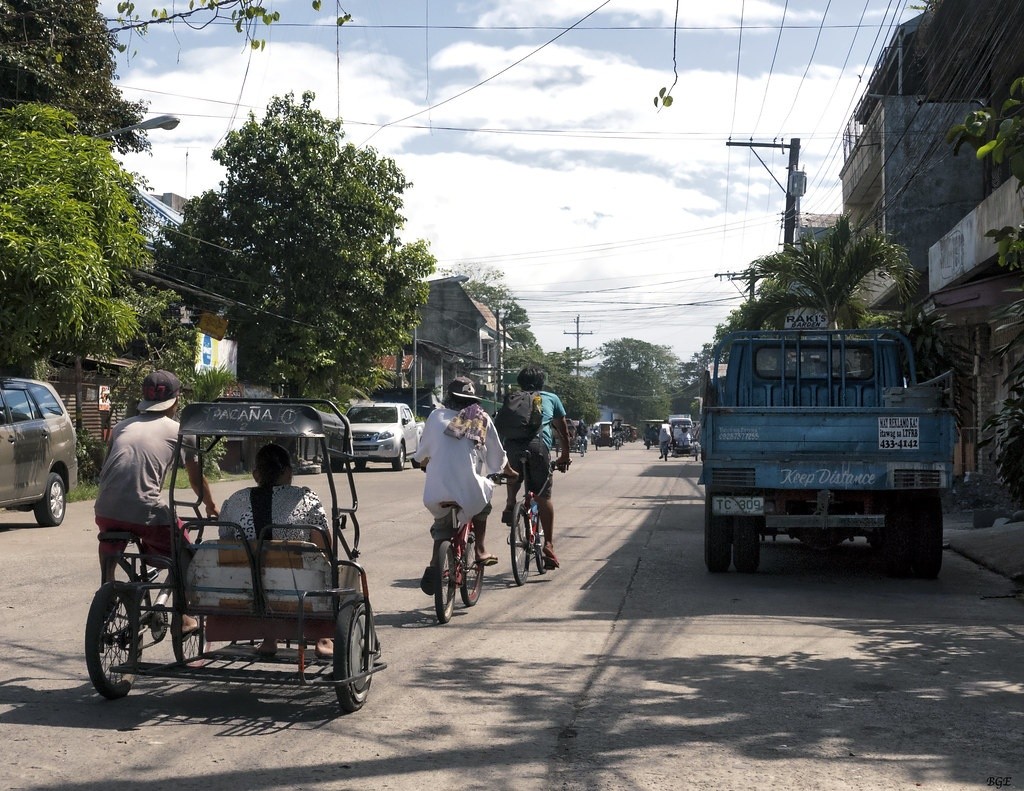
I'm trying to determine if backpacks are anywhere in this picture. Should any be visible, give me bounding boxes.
[494,390,542,440]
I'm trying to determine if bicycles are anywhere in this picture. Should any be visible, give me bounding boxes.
[492,458,572,586]
[410,456,521,623]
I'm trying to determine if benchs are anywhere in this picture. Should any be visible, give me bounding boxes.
[190,541,339,612]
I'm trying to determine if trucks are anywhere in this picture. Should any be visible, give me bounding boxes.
[697,326,964,581]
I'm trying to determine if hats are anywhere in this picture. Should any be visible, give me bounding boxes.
[137,370,178,412]
[449,376,482,400]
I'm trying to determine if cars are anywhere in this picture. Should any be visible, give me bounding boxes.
[590,421,615,445]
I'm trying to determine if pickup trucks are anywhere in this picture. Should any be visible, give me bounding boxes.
[345,402,426,471]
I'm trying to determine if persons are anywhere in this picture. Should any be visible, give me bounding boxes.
[94,370,219,645]
[218,444,334,661]
[410,376,519,595]
[642,424,651,445]
[659,419,673,459]
[496,367,570,567]
[694,419,702,438]
[615,422,624,446]
[575,419,587,453]
[625,426,631,443]
[678,427,691,445]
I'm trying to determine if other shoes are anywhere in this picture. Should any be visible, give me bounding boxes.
[665,458,667,461]
[659,456,663,459]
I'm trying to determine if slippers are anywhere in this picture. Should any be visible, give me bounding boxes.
[502,509,512,523]
[182,617,201,641]
[421,566,437,596]
[481,554,498,566]
[541,556,560,568]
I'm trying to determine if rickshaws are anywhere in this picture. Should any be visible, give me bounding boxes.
[555,419,588,457]
[640,420,664,450]
[594,423,626,450]
[657,418,699,462]
[84,395,388,714]
[620,423,637,443]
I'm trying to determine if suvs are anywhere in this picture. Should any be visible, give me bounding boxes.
[0,378,78,528]
